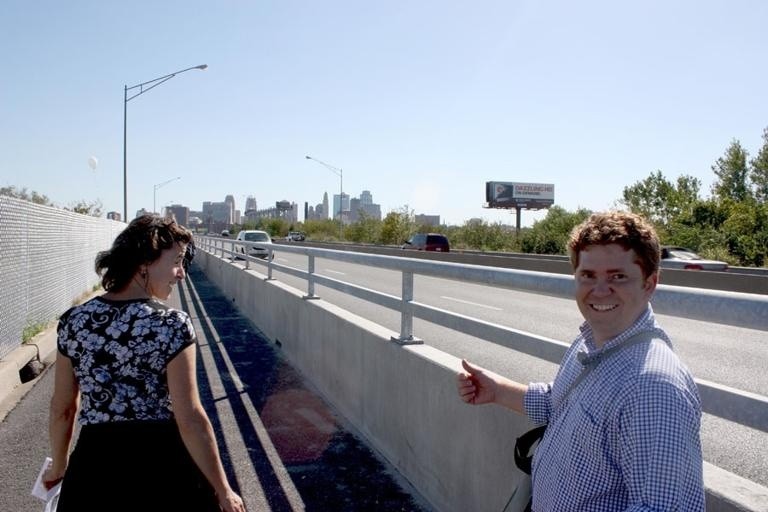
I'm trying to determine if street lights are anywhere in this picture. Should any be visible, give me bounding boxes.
[153,176,182,217]
[160,200,175,216]
[306,156,344,241]
[124,63,208,223]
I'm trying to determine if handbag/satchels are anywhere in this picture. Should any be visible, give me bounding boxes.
[501,472,533,511]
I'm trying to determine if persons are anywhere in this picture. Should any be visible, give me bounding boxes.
[29,209,251,512]
[454,204,714,512]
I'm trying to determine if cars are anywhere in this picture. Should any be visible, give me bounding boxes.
[221,229,229,237]
[659,245,729,272]
[285,230,304,241]
[233,230,275,261]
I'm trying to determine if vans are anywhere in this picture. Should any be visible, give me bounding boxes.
[400,233,449,252]
